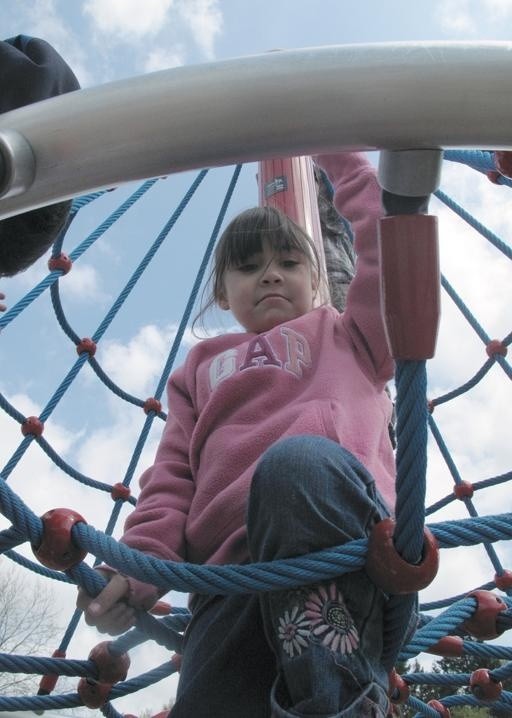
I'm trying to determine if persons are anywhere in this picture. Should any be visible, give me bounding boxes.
[76,152,405,718]
